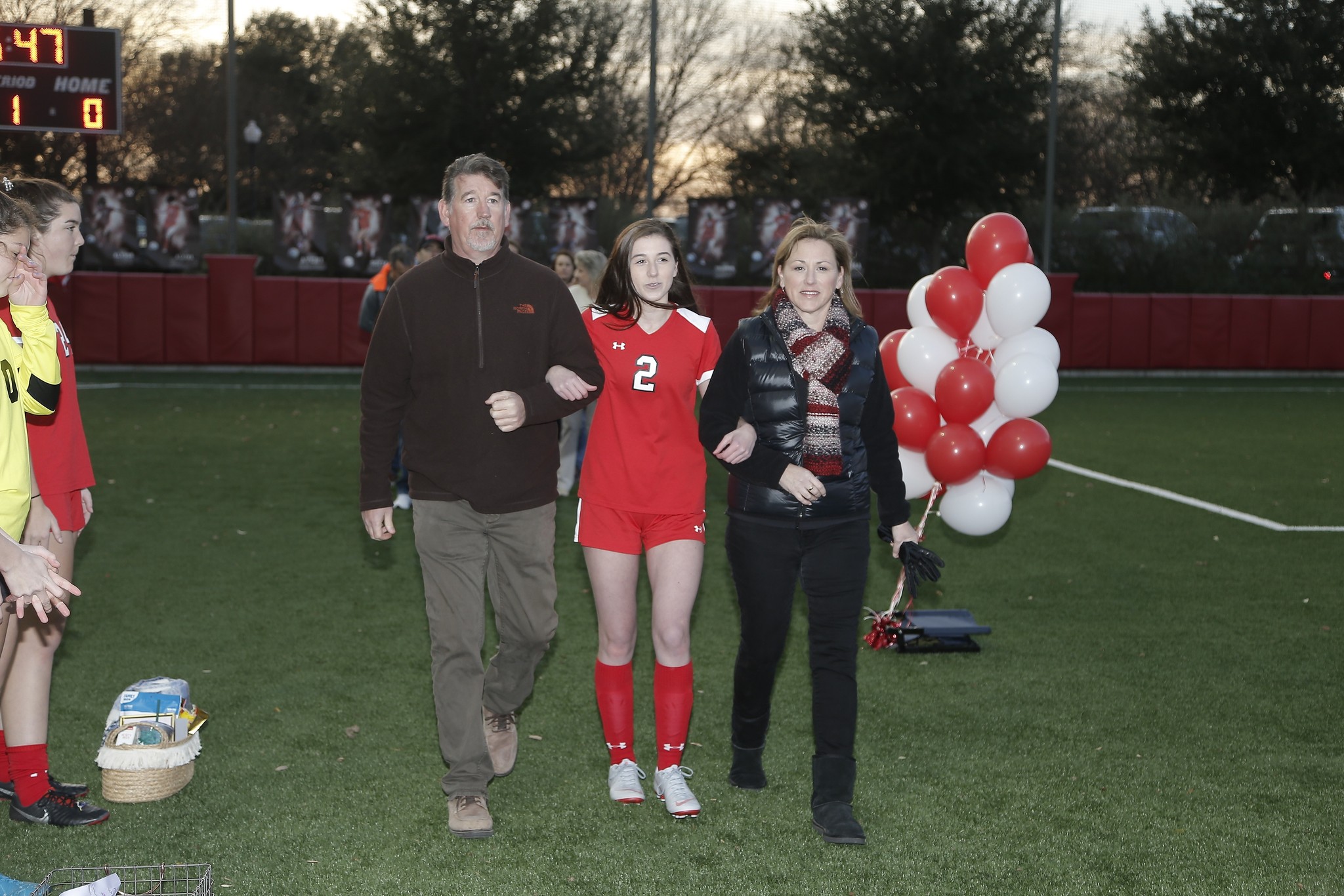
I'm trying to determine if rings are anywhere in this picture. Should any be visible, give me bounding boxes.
[808,484,814,491]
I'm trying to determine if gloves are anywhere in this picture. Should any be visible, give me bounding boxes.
[878,522,945,600]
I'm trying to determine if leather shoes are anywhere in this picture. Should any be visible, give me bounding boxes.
[447,795,494,839]
[482,705,519,778]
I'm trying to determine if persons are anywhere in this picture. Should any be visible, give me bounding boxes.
[698,211,920,845]
[94,186,857,280]
[1,530,84,626]
[546,249,587,475]
[508,239,521,254]
[553,249,610,501]
[357,243,420,509]
[2,176,111,826]
[1,189,62,649]
[486,216,828,818]
[358,154,605,837]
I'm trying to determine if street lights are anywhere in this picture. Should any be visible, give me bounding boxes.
[244,119,263,218]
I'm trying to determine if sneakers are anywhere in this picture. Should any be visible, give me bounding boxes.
[0,774,89,803]
[653,763,701,819]
[607,758,647,804]
[9,790,111,827]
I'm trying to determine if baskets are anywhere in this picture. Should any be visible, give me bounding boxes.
[94,721,203,804]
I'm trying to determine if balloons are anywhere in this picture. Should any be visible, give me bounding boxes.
[878,208,1061,536]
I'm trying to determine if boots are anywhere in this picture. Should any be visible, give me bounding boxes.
[728,740,768,789]
[809,753,868,845]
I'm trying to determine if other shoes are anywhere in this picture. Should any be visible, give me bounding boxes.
[392,494,413,510]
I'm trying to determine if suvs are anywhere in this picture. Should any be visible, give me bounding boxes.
[1062,202,1209,255]
[1242,205,1340,291]
